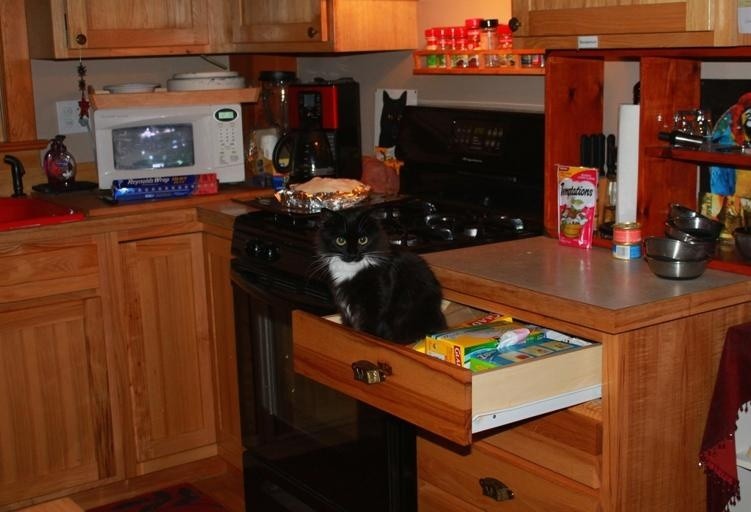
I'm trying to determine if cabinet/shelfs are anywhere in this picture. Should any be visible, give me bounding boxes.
[3,224,237,512]
[228,235,750,512]
[221,0,418,54]
[537,48,751,280]
[511,1,751,60]
[25,1,220,62]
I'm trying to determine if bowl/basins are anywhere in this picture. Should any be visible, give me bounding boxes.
[644,202,725,281]
[732,226,751,259]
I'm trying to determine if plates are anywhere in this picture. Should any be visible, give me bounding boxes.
[103,82,160,95]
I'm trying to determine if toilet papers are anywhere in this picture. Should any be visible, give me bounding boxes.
[615,104,639,223]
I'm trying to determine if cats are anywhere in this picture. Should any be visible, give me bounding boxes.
[312,202,450,347]
[377,89,409,148]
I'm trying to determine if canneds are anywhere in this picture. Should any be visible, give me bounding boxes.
[612,223,643,243]
[612,243,643,259]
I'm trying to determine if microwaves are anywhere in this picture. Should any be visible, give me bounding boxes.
[90,103,246,190]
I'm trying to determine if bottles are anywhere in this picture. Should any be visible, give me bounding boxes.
[480,18,499,68]
[657,131,706,148]
[43,134,77,191]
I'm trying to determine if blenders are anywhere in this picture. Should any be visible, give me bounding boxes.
[259,70,296,130]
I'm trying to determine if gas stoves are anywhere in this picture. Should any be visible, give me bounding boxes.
[232,190,545,285]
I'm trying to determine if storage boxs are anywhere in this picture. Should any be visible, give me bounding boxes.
[423,312,579,372]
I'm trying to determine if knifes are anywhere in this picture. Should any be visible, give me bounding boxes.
[577,131,615,180]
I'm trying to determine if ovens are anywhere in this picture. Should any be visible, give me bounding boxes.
[228,267,417,510]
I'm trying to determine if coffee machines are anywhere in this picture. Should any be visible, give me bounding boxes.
[276,77,362,183]
